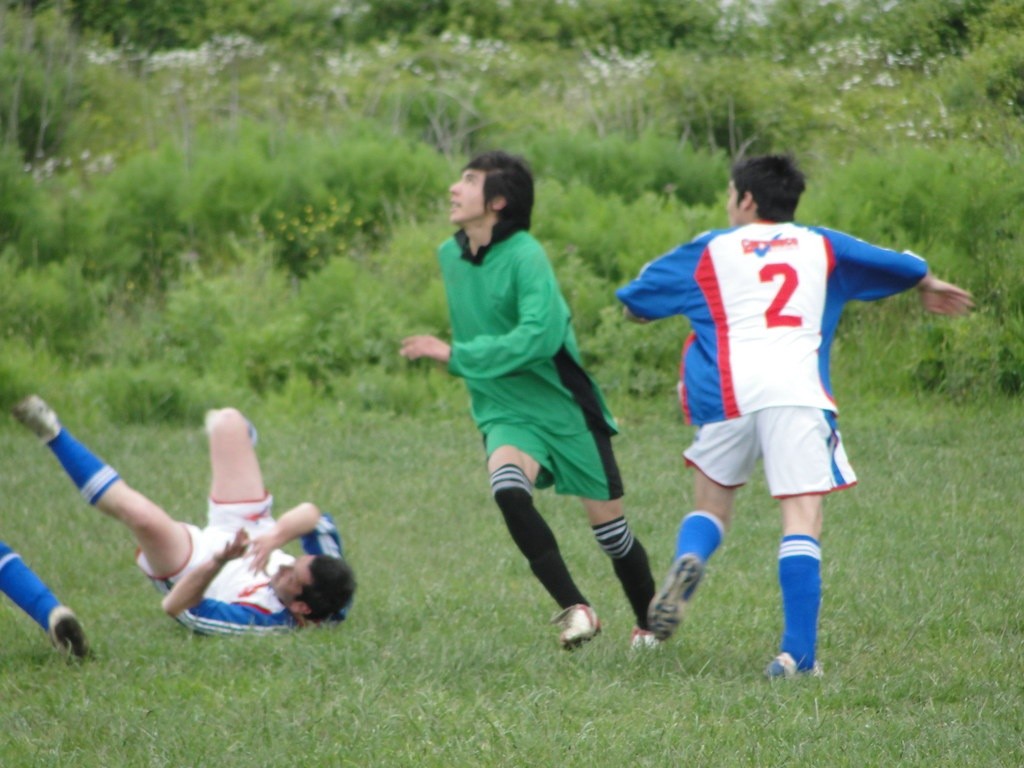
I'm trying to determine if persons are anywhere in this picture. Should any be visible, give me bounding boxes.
[617,154,975,681]
[399,152,664,651]
[12,392,357,639]
[0,541,91,657]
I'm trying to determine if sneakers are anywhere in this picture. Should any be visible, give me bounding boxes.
[557,602,601,650]
[11,393,59,440]
[646,552,705,641]
[762,652,824,680]
[49,604,89,664]
[630,623,663,650]
